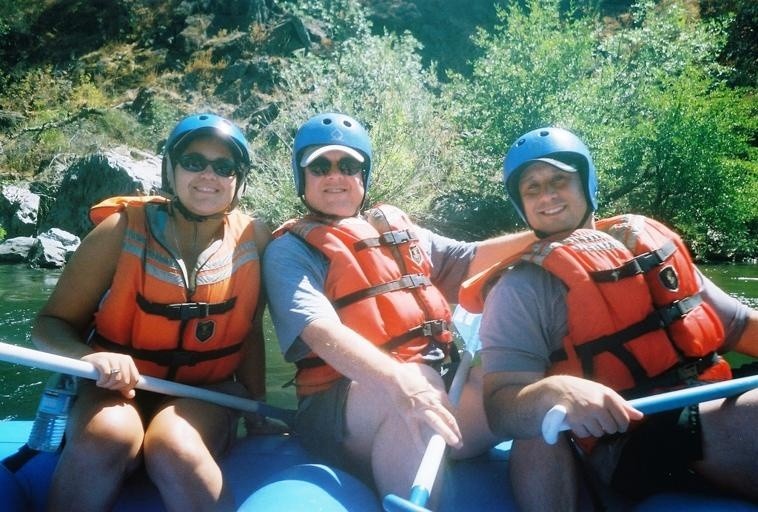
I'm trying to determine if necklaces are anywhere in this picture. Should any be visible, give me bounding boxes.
[166,213,224,255]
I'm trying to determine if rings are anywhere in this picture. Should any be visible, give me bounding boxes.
[111,369,120,374]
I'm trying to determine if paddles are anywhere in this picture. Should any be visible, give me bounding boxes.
[382,306,483,512]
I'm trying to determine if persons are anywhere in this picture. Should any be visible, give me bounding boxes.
[31,113,273,512]
[479,127,758,512]
[262,114,542,502]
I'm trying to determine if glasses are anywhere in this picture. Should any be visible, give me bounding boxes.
[307,156,362,176]
[175,151,244,177]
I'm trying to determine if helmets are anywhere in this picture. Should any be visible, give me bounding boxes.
[502,127,600,226]
[291,112,373,196]
[161,114,251,183]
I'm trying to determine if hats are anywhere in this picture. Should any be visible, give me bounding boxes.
[525,158,580,174]
[172,126,244,160]
[299,144,365,168]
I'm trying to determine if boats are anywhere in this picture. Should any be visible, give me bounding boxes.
[0,412,754,512]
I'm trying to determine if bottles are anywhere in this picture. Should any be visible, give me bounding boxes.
[26,370,79,454]
